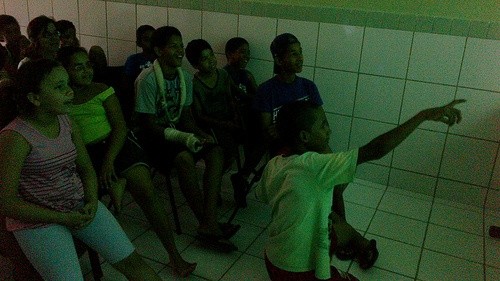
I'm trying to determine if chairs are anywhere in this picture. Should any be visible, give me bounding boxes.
[0,68,246,281]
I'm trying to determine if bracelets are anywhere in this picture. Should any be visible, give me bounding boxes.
[424,108,430,120]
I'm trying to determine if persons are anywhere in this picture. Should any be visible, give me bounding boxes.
[55,45,198,277]
[222,35,262,135]
[259,97,468,281]
[131,24,242,255]
[184,39,264,206]
[0,57,163,281]
[256,32,379,270]
[0,14,159,130]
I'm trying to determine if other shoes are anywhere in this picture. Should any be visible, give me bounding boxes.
[231,173,250,206]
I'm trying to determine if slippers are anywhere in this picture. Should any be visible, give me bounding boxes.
[195,233,237,253]
[335,243,357,260]
[358,239,379,270]
[220,222,240,240]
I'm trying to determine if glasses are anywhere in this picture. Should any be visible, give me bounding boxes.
[36,30,61,39]
[69,62,93,72]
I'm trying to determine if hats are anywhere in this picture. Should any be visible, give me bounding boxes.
[270,33,300,74]
